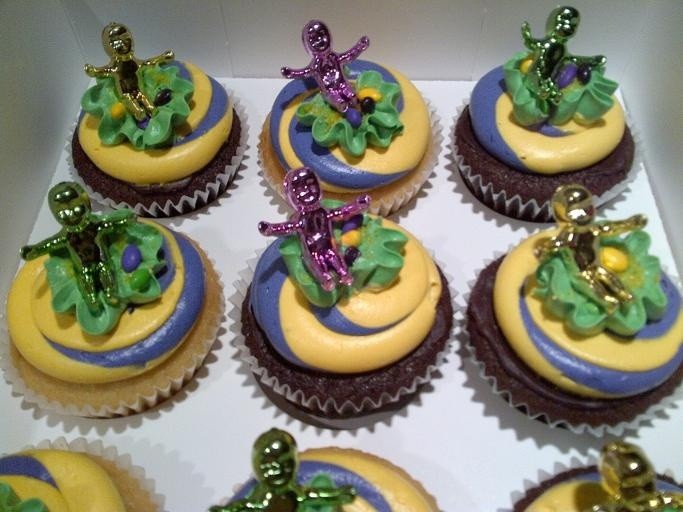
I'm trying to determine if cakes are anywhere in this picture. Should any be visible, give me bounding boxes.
[208,427,443,512]
[64,23,250,217]
[448,6,645,222]
[257,19,444,217]
[0,181,226,418]
[461,184,683,437]
[230,168,460,420]
[512,440,683,512]
[0,437,169,512]
